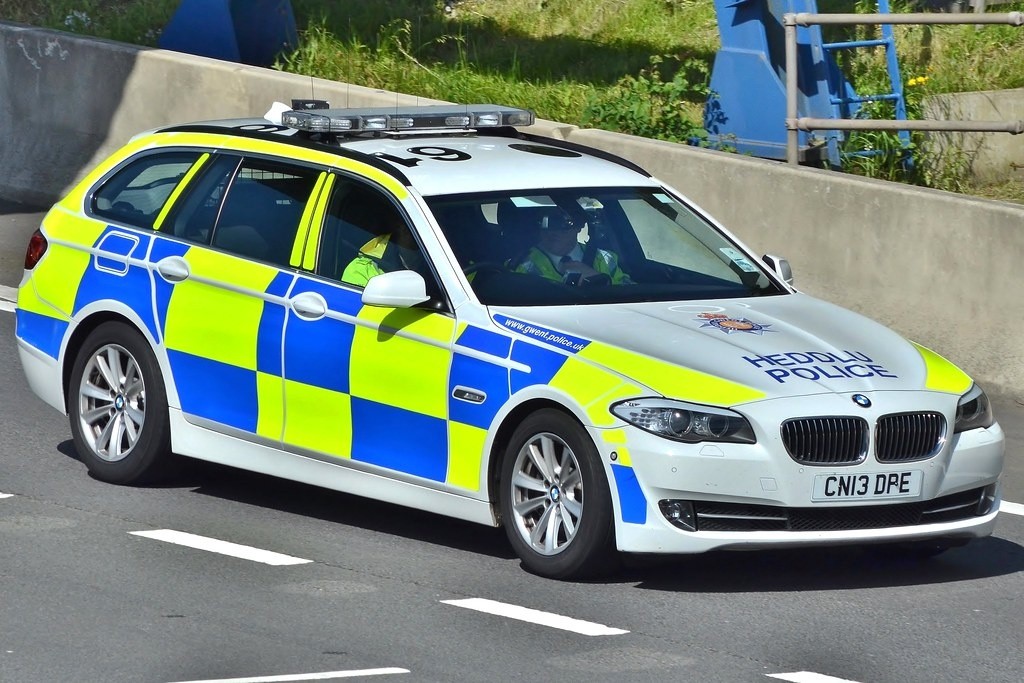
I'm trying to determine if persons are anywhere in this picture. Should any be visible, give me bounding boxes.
[505,219,636,285]
[340,218,477,289]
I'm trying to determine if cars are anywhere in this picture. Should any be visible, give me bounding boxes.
[15,97,1007,580]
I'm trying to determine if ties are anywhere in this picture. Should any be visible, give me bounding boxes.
[561,256,570,262]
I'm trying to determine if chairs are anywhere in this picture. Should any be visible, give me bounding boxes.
[208,182,291,267]
[344,211,402,282]
